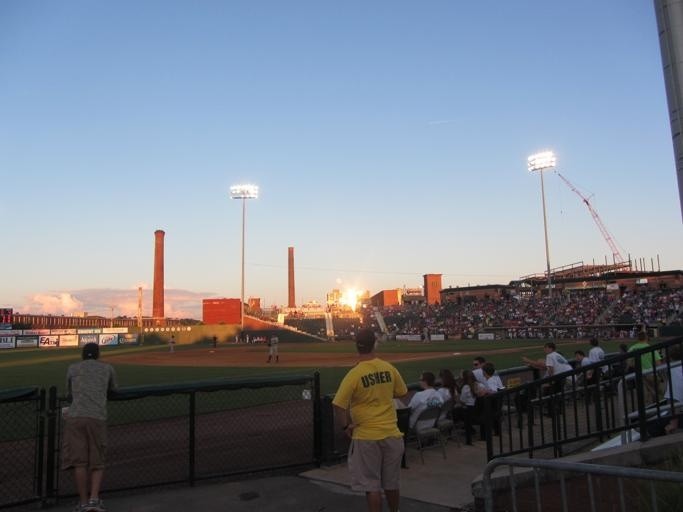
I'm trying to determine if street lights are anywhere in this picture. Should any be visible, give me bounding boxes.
[527,148,557,301]
[229,183,260,334]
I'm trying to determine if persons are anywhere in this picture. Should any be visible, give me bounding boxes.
[522,331,683,401]
[168,336,176,352]
[347,288,683,338]
[266,332,279,363]
[64,343,117,512]
[408,356,504,430]
[330,329,409,512]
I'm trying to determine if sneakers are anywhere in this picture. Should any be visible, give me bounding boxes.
[76,498,107,511]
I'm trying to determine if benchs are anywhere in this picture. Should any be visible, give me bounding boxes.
[530,375,625,418]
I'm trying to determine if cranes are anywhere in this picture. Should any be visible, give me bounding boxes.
[558,173,629,270]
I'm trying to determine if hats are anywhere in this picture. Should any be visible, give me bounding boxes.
[83,343,99,357]
[356,329,375,354]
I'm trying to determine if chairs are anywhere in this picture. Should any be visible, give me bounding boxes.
[464,397,492,432]
[410,406,446,464]
[433,399,465,449]
[489,392,504,436]
